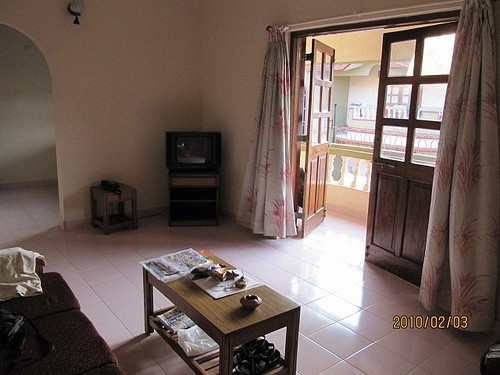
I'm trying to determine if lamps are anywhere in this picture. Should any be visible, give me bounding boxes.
[68,0,83,25]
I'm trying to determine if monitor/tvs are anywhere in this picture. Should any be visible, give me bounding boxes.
[165,131,222,172]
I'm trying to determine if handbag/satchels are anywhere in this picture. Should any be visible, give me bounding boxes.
[0,308,54,362]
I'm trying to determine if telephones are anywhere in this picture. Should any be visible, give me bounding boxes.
[100,179,121,199]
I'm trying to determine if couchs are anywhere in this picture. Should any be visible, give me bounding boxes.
[0,246,126,375]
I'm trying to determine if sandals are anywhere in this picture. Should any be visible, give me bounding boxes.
[236,343,281,374]
[233,335,269,365]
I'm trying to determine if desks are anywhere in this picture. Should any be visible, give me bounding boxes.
[90,182,138,235]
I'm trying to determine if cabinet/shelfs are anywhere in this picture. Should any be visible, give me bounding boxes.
[168,174,220,226]
